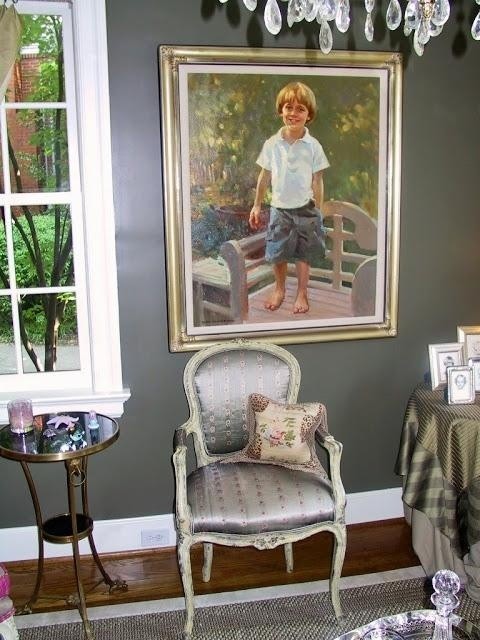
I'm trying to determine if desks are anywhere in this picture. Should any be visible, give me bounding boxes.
[193,254,275,326]
[0,411,127,640]
[393,381,479,605]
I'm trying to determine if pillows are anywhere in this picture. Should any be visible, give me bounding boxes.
[220,395,328,479]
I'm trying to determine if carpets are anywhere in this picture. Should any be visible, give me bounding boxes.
[0,566,480,640]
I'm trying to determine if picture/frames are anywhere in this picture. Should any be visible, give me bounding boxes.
[157,44,405,353]
[428,325,480,404]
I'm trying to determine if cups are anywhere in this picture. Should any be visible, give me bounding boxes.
[10,436,36,455]
[6,399,34,434]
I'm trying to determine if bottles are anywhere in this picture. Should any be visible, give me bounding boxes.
[88,411,100,431]
[90,428,100,446]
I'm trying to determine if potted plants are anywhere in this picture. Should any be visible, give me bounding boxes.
[213,90,274,259]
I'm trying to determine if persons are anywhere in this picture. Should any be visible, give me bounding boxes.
[249,80,332,313]
[444,356,454,367]
[455,374,466,390]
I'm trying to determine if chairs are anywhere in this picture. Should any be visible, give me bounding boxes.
[169,339,348,640]
[221,201,377,324]
